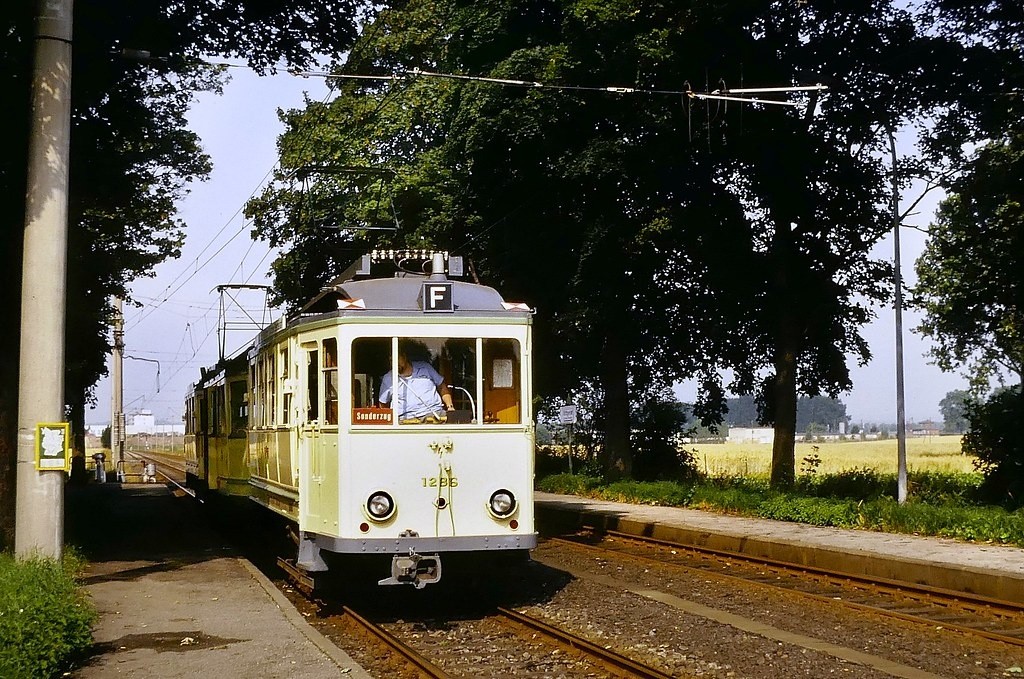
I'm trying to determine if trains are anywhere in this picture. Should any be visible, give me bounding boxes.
[183,168,539,598]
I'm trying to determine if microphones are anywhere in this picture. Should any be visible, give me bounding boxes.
[446,385,477,423]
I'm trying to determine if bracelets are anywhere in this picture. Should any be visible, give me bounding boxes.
[447,404,453,408]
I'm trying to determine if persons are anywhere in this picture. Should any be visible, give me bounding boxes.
[379,346,455,420]
